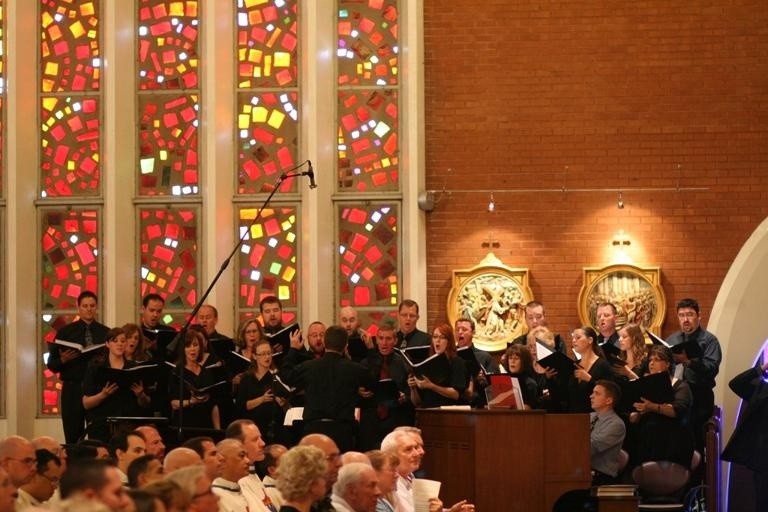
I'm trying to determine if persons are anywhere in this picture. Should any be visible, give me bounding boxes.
[558,380,626,511]
[720,360,768,512]
[542,326,616,414]
[605,323,652,383]
[2,418,475,512]
[665,296,722,481]
[616,343,695,473]
[49,293,570,456]
[595,299,626,363]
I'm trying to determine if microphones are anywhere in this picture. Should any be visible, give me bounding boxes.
[305,161,317,190]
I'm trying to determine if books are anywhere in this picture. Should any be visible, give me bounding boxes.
[611,353,639,381]
[533,335,586,385]
[645,329,699,365]
[571,340,622,363]
[611,370,675,434]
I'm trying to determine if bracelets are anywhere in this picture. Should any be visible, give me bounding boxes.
[657,403,661,413]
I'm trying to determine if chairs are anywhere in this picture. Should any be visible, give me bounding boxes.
[631,459,696,512]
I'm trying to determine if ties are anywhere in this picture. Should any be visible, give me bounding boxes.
[84,325,93,347]
[400,335,408,353]
[378,356,391,420]
[590,415,599,433]
[671,334,685,384]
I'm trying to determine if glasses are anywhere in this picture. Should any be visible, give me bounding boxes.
[7,457,38,465]
[42,474,60,485]
[325,456,341,461]
[256,353,271,356]
[379,468,398,474]
